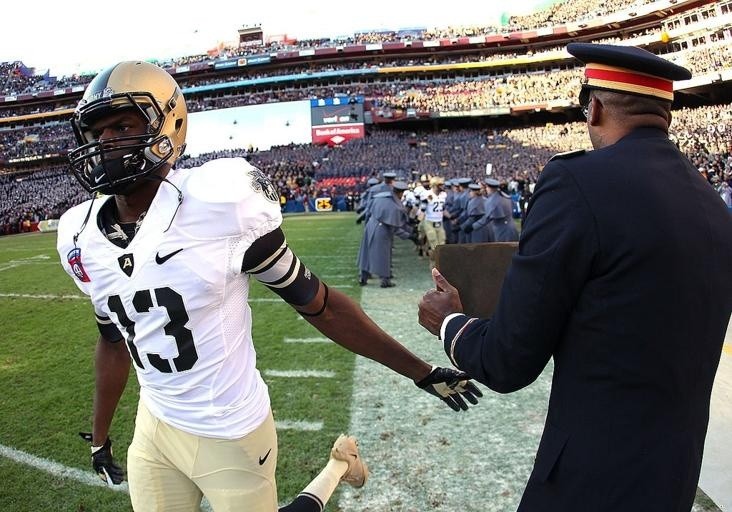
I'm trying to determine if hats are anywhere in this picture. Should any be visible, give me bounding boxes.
[368,173,410,193]
[566,42,692,106]
[445,177,499,191]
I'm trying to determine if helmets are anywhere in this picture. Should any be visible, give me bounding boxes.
[68,61,187,194]
[413,174,443,189]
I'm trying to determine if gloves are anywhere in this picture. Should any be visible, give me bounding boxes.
[450,214,473,233]
[80,430,127,490]
[409,218,420,240]
[415,366,483,412]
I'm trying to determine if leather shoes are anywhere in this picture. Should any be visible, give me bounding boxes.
[359,278,367,284]
[381,283,395,287]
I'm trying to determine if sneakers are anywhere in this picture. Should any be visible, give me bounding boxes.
[331,434,365,489]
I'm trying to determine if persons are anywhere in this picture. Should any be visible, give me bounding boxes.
[414,40,732,511]
[0,61,104,236]
[151,1,594,230]
[356,170,520,288]
[594,1,732,210]
[57,58,483,511]
